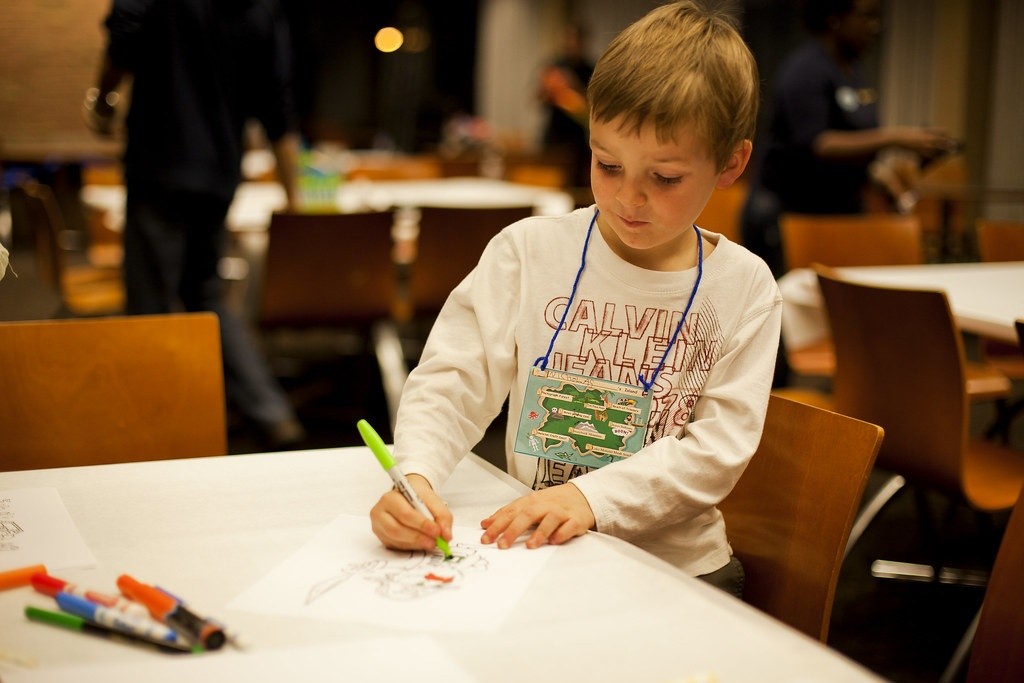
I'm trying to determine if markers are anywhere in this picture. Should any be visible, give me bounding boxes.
[355,419,453,559]
[54,593,176,643]
[28,572,147,621]
[116,574,227,652]
[0,564,47,593]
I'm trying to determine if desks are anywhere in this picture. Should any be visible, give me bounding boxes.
[0,446,887,683]
[227,176,577,280]
[832,262,1024,350]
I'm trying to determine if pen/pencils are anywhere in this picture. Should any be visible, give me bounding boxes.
[156,586,251,650]
[24,607,204,655]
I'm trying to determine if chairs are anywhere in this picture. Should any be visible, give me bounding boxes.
[810,259,1024,631]
[974,219,1024,261]
[718,393,883,648]
[227,203,407,446]
[0,314,226,472]
[390,203,533,401]
[939,481,1024,683]
[14,179,126,318]
[779,215,923,274]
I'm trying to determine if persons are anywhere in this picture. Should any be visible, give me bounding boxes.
[369,0,782,600]
[737,1,959,388]
[88,0,304,452]
[531,51,596,189]
